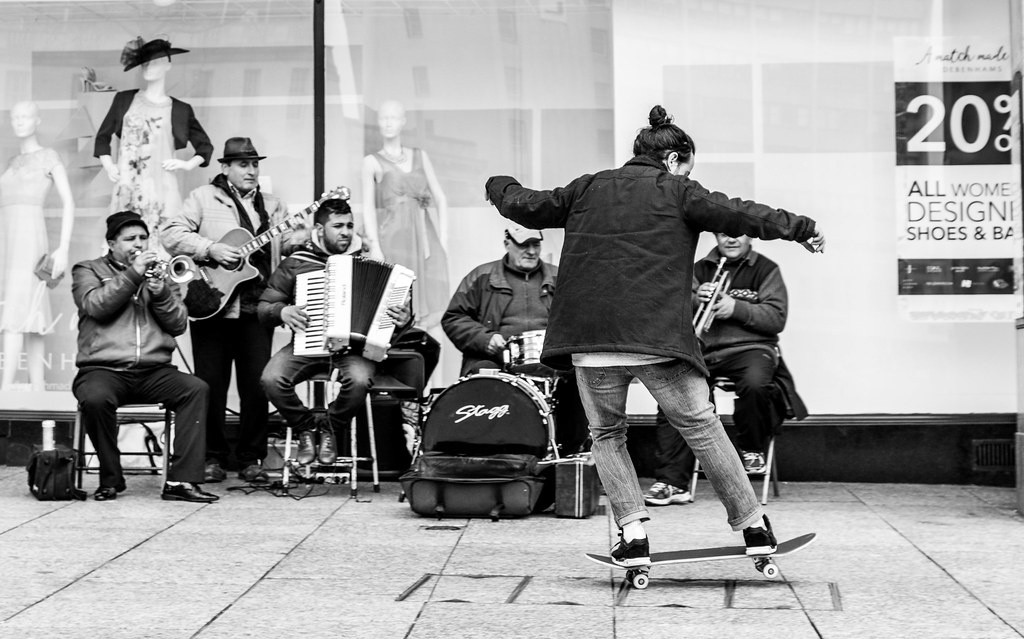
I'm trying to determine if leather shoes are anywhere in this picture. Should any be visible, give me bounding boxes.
[318,430,338,463]
[94,479,127,501]
[296,426,318,464]
[161,481,219,501]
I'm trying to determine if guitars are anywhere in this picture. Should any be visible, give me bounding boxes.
[180,185,352,323]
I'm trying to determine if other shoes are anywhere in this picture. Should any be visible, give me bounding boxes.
[238,464,269,482]
[204,463,227,481]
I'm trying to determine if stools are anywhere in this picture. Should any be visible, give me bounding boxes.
[688,378,780,505]
[282,375,381,496]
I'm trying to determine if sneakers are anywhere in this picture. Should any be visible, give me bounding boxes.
[743,514,777,556]
[643,482,690,505]
[741,451,767,474]
[609,533,651,567]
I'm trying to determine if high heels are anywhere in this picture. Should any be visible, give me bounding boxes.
[80,67,117,92]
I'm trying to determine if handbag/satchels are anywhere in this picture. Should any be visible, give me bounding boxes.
[26,448,87,502]
[32,253,64,289]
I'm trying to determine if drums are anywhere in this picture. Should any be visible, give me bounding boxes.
[422,371,558,460]
[501,328,574,377]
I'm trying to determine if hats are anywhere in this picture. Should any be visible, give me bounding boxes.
[106,211,150,240]
[120,36,190,72]
[504,222,544,244]
[217,136,267,163]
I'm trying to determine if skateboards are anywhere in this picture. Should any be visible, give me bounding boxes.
[583,532,818,591]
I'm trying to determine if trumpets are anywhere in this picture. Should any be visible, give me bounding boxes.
[134,249,198,284]
[691,256,732,357]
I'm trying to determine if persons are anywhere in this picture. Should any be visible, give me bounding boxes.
[71,212,218,502]
[440,224,559,395]
[0,98,75,396]
[92,39,215,264]
[642,230,809,506]
[484,105,825,568]
[159,137,291,479]
[359,101,451,333]
[257,199,414,465]
